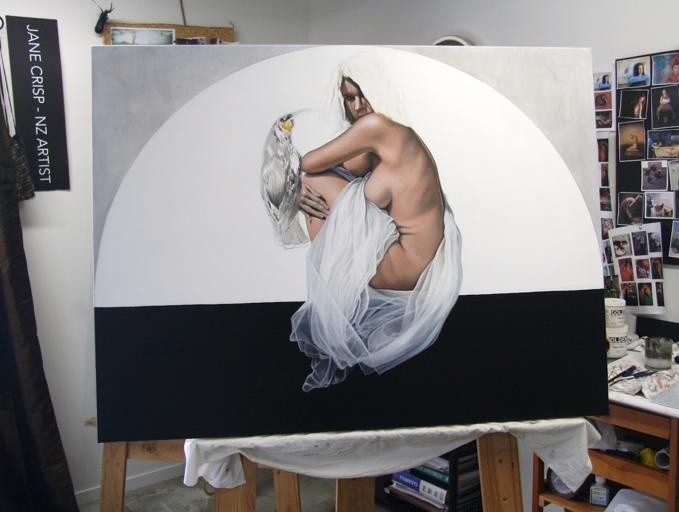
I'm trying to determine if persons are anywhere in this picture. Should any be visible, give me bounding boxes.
[656,89,675,122]
[628,63,649,86]
[599,74,610,90]
[296,76,446,292]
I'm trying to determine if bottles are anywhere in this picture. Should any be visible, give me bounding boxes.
[588,476,609,507]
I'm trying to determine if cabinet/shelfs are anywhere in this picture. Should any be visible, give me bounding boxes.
[522,341,679,511]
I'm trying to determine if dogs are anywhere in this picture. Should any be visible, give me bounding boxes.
[654,204,673,217]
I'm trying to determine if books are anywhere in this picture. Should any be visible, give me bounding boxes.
[385,449,480,511]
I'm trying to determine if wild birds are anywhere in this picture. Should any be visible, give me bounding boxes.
[260,113,310,250]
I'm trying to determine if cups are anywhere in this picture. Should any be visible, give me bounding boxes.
[645,336,673,372]
